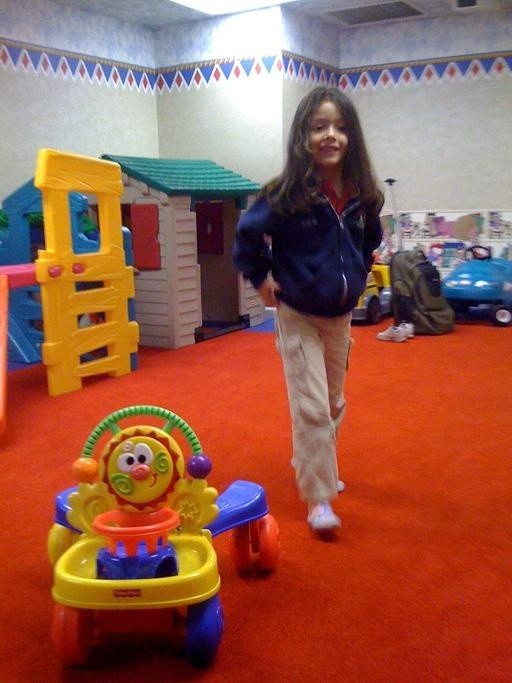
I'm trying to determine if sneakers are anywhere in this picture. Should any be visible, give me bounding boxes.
[376,322,415,343]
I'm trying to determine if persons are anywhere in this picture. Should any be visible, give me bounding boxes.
[230,84,385,534]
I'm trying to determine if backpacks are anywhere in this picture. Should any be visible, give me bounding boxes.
[390,249,455,334]
